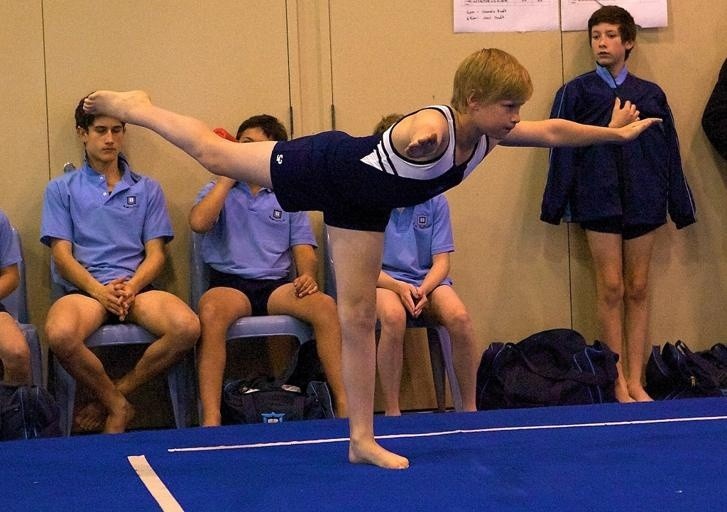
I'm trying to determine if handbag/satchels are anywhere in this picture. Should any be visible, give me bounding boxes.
[476,329,727,411]
[0,384,61,441]
[220,378,335,425]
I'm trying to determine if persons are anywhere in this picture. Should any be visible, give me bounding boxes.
[373,114,476,416]
[187,113,348,427]
[702,57,727,161]
[1,210,33,387]
[38,91,201,435]
[82,49,664,470]
[539,5,698,404]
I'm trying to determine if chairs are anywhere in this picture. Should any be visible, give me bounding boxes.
[189,228,313,429]
[48,255,188,438]
[323,224,462,414]
[0,225,43,437]
